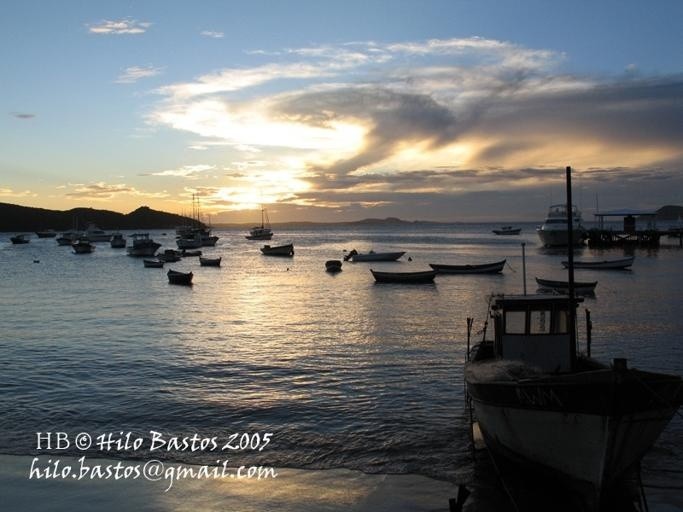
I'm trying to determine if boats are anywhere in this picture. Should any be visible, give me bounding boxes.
[430,261,505,274]
[464,289,683,511]
[492,227,521,235]
[536,203,585,248]
[560,258,633,269]
[370,268,439,284]
[325,259,342,274]
[351,250,405,262]
[9,194,222,286]
[536,277,597,296]
[260,243,293,255]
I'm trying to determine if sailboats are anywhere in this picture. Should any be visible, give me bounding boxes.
[245,208,274,240]
